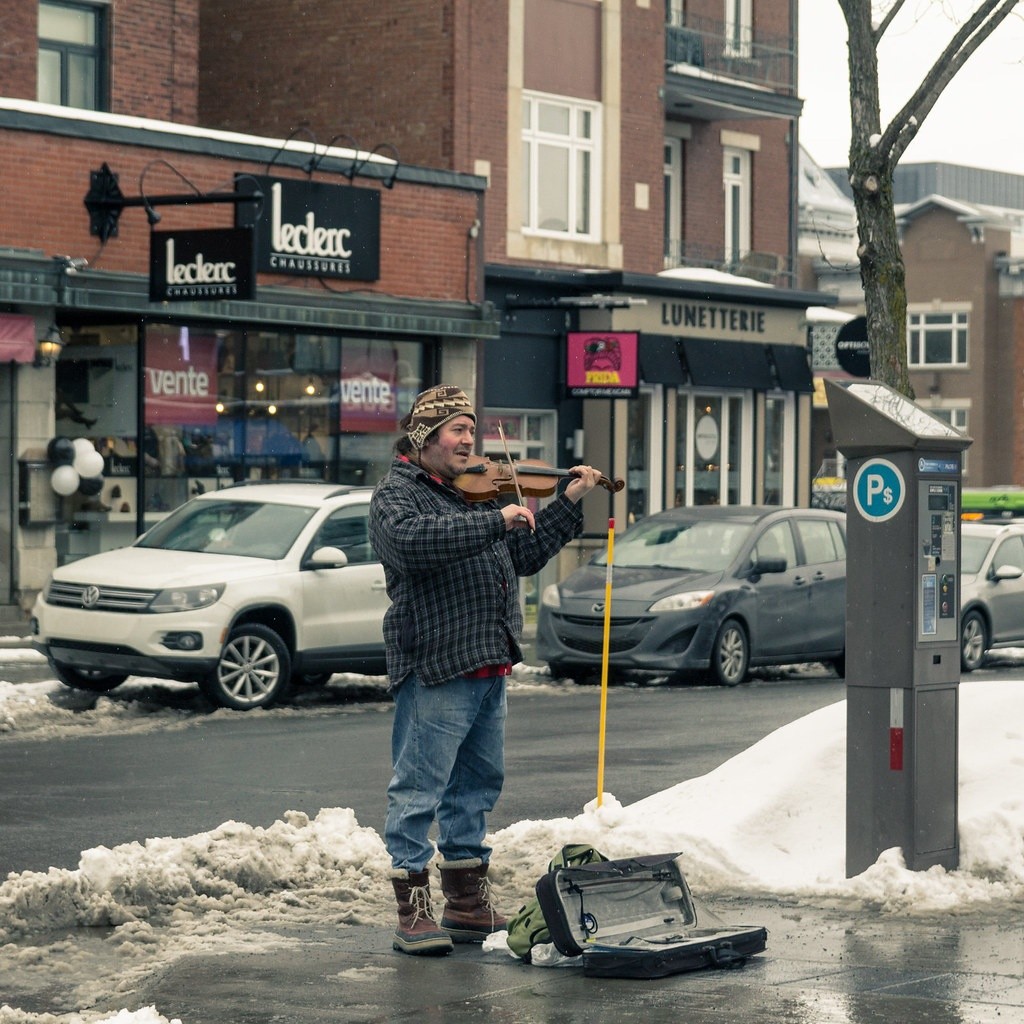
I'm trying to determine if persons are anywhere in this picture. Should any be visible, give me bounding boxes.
[368,383,602,953]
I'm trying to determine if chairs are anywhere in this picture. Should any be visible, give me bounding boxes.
[729,530,779,555]
[263,507,322,557]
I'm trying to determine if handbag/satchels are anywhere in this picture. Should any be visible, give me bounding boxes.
[531,941,584,967]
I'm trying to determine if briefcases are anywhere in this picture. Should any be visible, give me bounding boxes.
[536,852,767,979]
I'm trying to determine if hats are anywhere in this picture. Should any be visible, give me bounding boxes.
[400,384,477,468]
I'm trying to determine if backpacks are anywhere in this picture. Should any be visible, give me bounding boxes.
[507,843,611,962]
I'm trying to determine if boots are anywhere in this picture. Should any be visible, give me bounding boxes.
[388,868,454,954]
[436,858,508,942]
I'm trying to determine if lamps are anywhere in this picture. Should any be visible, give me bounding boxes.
[33,324,65,370]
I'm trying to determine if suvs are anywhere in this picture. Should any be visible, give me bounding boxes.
[27,479,394,713]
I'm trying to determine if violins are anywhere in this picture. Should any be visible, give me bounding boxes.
[453,454,625,503]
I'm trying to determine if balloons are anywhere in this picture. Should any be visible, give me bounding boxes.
[48,435,106,498]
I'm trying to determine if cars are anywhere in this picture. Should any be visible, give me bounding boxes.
[536,504,846,688]
[961,515,1023,674]
